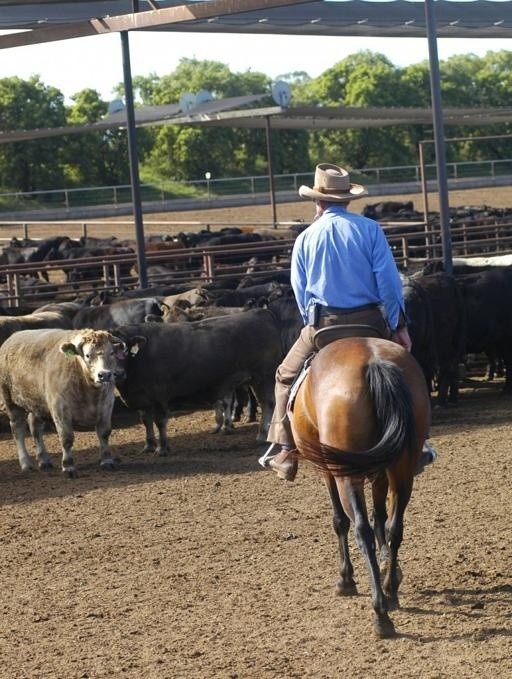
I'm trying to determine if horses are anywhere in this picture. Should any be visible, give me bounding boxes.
[290,334,433,640]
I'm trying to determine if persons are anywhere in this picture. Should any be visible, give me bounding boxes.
[258,163,437,482]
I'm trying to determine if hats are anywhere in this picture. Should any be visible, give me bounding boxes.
[299,163,369,203]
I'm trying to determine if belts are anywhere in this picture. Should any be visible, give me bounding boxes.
[318,303,375,316]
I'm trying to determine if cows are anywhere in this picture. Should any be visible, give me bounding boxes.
[1,199,512,479]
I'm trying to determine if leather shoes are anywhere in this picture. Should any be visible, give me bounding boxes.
[258,450,298,482]
[414,450,437,475]
